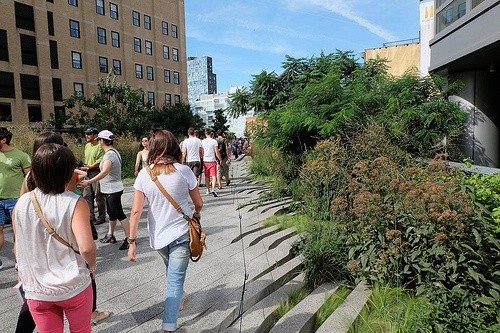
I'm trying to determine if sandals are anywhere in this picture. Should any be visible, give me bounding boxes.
[91,310,110,325]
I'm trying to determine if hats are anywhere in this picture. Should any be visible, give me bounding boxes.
[94,129,115,140]
[188,128,194,133]
[84,127,99,134]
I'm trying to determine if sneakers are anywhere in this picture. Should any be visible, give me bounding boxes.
[119,238,137,250]
[99,234,117,244]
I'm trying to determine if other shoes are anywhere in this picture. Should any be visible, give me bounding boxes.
[211,189,218,197]
[93,219,106,225]
[0,259,19,270]
[199,184,206,187]
[206,190,210,194]
[225,180,230,186]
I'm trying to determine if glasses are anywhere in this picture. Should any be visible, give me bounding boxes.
[96,138,103,142]
[141,140,147,142]
[86,132,92,135]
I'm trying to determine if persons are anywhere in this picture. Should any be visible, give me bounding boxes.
[176,127,231,198]
[12,143,96,333]
[82,127,107,224]
[12,132,111,333]
[127,129,203,333]
[77,129,136,250]
[232,135,251,160]
[0,126,31,271]
[135,135,151,176]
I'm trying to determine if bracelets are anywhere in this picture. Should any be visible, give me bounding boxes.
[194,208,200,214]
[127,237,135,243]
[89,262,97,273]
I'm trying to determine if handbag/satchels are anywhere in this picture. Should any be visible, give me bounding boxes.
[182,213,206,262]
[189,162,202,176]
